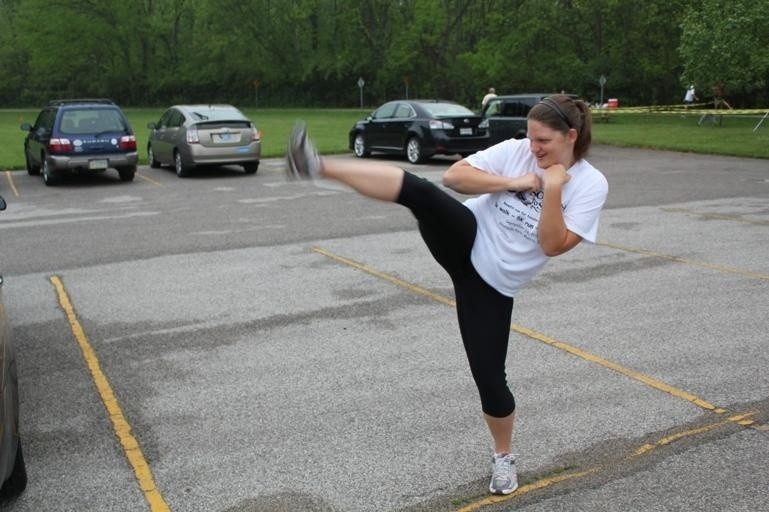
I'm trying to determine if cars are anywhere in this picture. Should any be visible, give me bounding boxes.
[347,98,492,165]
[146,101,262,177]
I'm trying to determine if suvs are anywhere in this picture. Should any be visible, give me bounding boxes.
[19,97,139,187]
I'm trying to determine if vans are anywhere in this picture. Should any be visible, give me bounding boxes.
[482,91,579,146]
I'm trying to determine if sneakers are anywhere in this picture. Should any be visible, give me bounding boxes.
[488,452,519,495]
[284,119,320,182]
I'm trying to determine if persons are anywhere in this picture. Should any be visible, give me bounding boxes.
[481,88,500,113]
[285,93,608,496]
[682,84,699,119]
